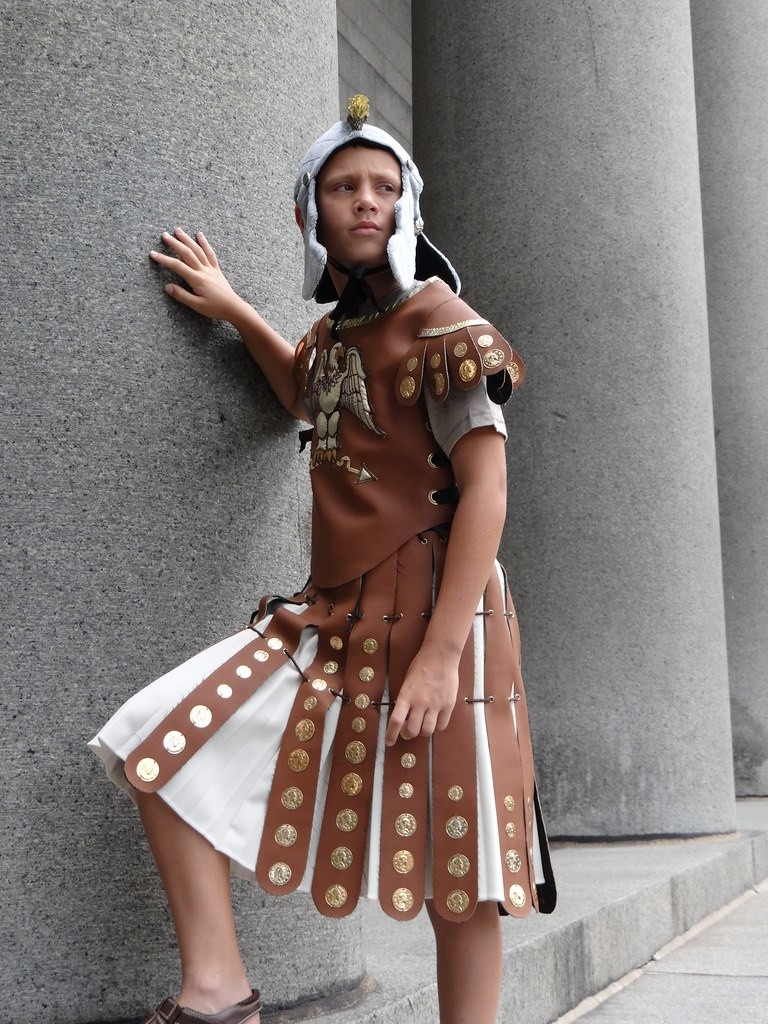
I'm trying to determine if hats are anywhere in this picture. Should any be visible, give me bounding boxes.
[279,93,462,305]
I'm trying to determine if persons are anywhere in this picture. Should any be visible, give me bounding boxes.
[86,97,557,1024]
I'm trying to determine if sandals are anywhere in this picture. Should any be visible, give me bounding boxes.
[119,989,263,1024]
